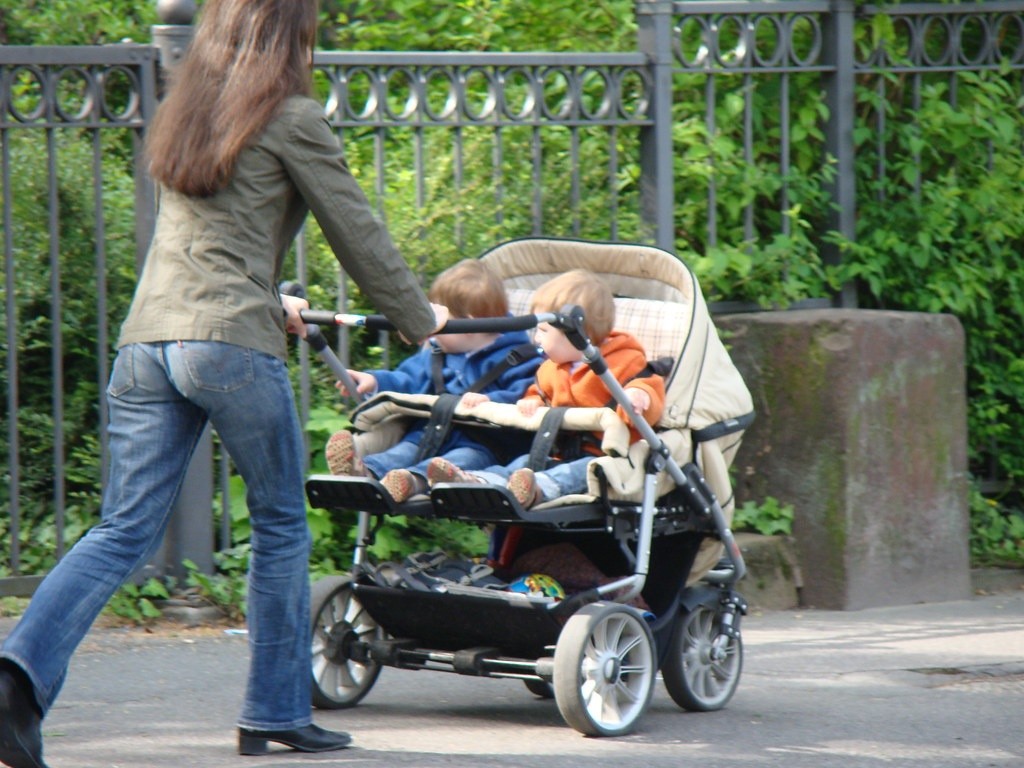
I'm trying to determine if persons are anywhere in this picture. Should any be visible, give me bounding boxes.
[427,268,666,511]
[0,0,455,768]
[325,258,548,503]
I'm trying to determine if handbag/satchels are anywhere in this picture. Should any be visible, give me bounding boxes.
[374,550,509,591]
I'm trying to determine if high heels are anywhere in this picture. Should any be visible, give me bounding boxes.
[238,722,351,754]
[0,662,49,768]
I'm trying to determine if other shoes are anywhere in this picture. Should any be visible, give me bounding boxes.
[427,458,478,493]
[378,468,414,502]
[505,468,537,507]
[325,430,367,475]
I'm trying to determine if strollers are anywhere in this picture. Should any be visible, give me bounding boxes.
[280,235,751,738]
